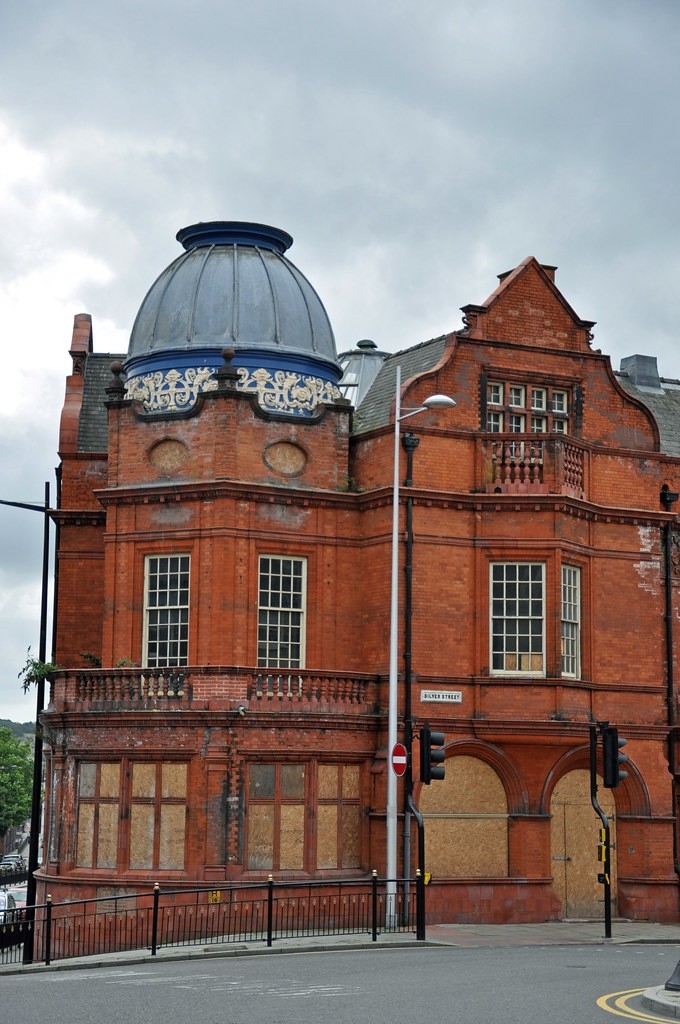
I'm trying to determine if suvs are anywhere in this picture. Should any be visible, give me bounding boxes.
[0,889,17,923]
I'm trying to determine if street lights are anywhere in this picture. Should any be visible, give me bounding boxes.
[386,394,455,929]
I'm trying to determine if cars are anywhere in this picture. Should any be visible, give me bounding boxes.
[0,854,27,872]
[7,889,27,920]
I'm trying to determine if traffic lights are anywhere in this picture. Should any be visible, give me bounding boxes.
[420,723,445,784]
[602,727,628,789]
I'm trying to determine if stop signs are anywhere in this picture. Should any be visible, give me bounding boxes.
[391,743,408,778]
